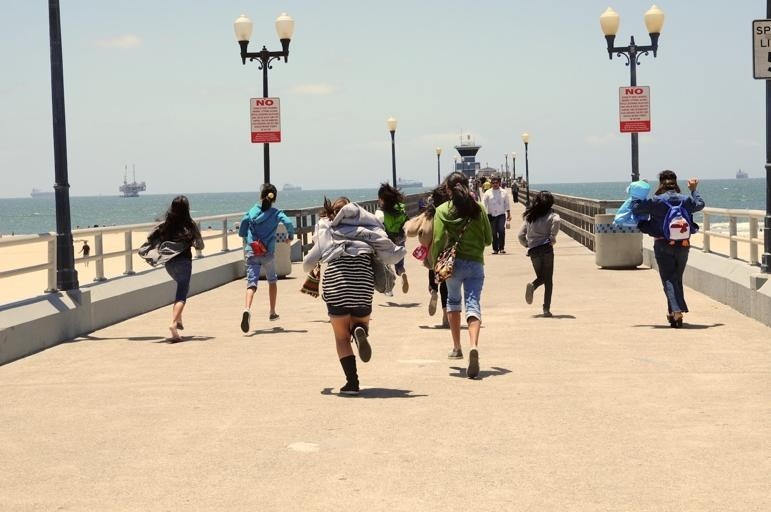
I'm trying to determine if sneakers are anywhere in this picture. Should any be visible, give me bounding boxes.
[353,326,372,362]
[384,290,394,297]
[169,321,185,341]
[270,311,281,319]
[544,312,553,317]
[341,382,360,394]
[448,349,463,358]
[492,248,507,254]
[401,272,410,294]
[429,292,449,328]
[525,283,535,304]
[240,309,251,332]
[467,348,480,377]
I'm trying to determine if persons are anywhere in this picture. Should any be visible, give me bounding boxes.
[78,241,91,269]
[303,197,406,393]
[517,190,561,316]
[367,167,527,328]
[428,172,494,378]
[238,184,295,333]
[630,169,705,328]
[138,195,205,342]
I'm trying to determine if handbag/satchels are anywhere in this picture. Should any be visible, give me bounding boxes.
[249,239,268,257]
[413,245,430,262]
[435,245,457,284]
[373,260,398,296]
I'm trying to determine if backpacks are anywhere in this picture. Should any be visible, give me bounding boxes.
[659,196,697,246]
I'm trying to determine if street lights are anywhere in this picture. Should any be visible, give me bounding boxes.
[385,115,403,199]
[231,7,292,282]
[451,153,458,197]
[598,4,664,247]
[484,151,517,190]
[434,146,446,195]
[518,131,534,212]
